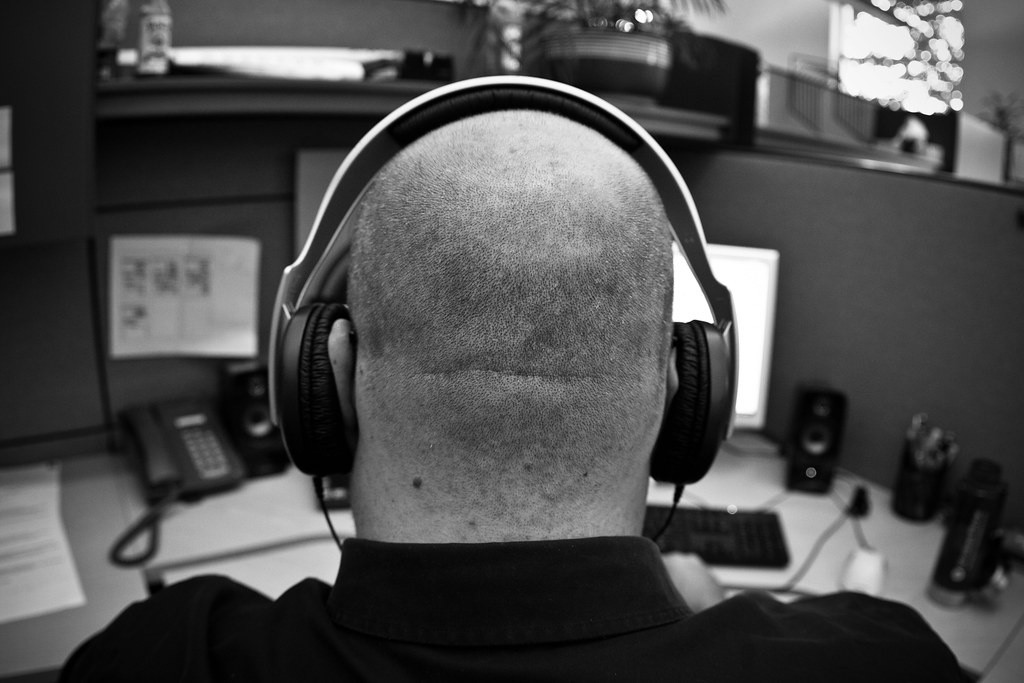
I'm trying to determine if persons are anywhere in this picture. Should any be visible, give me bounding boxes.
[3,96,990,683]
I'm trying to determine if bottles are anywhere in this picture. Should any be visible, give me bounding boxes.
[141,0,172,73]
[929,459,1006,604]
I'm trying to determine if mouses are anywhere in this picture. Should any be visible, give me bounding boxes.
[840,544,889,599]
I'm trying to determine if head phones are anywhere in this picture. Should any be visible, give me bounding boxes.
[261,72,739,491]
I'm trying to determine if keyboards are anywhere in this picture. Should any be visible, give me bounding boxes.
[642,504,789,569]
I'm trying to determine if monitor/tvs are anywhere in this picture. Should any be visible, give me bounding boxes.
[673,242,779,431]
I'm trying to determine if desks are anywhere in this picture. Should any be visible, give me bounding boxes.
[1,429,1024,681]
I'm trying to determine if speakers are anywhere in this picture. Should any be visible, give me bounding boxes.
[784,382,849,494]
[222,358,290,478]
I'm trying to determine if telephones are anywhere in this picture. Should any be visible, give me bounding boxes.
[120,397,243,507]
